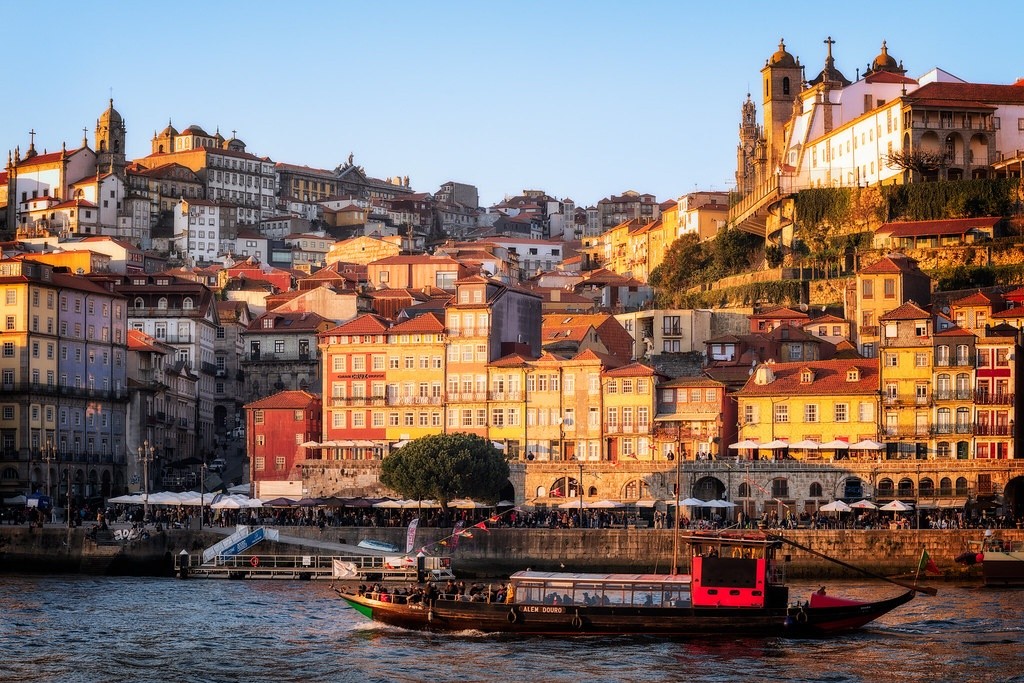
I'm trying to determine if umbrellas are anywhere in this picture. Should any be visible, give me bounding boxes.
[491,441,505,449]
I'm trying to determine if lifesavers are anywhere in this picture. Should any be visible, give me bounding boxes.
[796,611,808,623]
[572,616,583,630]
[506,610,516,623]
[251,557,259,566]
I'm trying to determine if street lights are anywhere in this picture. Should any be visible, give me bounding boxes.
[41,437,57,496]
[138,439,155,521]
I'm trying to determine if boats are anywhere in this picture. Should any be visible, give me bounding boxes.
[328,425,937,639]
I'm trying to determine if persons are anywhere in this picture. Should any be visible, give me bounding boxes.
[509,450,848,467]
[0,497,1023,602]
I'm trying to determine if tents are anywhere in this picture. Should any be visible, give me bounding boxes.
[107,482,914,525]
[300,439,409,459]
[729,440,887,459]
